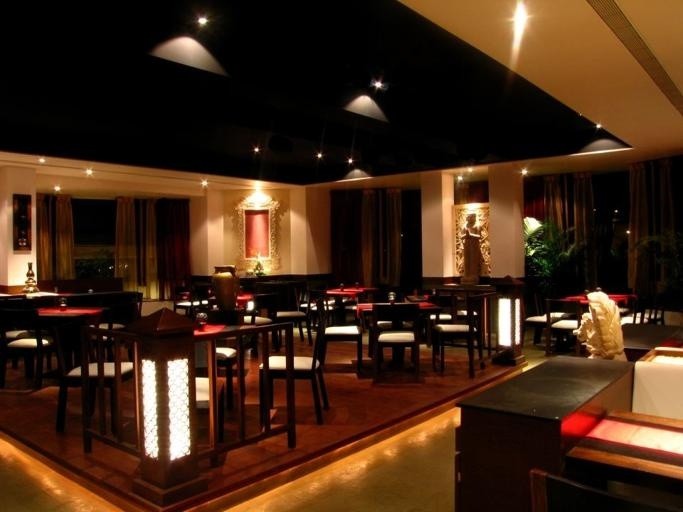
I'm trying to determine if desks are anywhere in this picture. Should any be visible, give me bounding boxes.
[453,356,635,511]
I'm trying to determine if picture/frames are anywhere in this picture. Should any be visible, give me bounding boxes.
[11,193,31,253]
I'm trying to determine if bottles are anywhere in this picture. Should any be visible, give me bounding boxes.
[195,314,207,330]
[56,295,69,311]
[386,289,397,305]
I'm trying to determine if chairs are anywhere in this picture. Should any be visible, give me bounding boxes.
[2,280,140,458]
[176,282,330,450]
[524,283,665,357]
[318,279,499,383]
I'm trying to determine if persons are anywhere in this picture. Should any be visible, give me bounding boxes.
[458,211,483,285]
